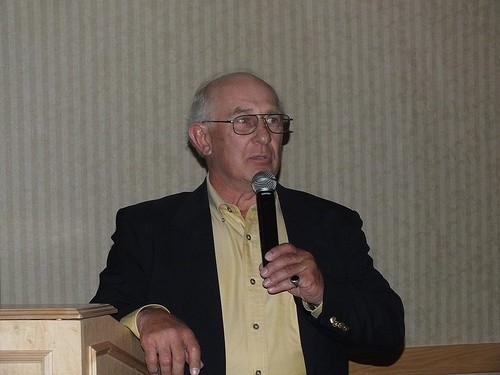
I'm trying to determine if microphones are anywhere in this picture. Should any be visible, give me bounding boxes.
[251,170,280,267]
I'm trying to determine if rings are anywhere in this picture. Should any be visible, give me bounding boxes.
[290,275,300,288]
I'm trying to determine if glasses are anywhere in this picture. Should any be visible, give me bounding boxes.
[202,113,294,135]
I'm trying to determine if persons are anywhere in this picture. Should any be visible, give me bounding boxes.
[89,71,405,375]
